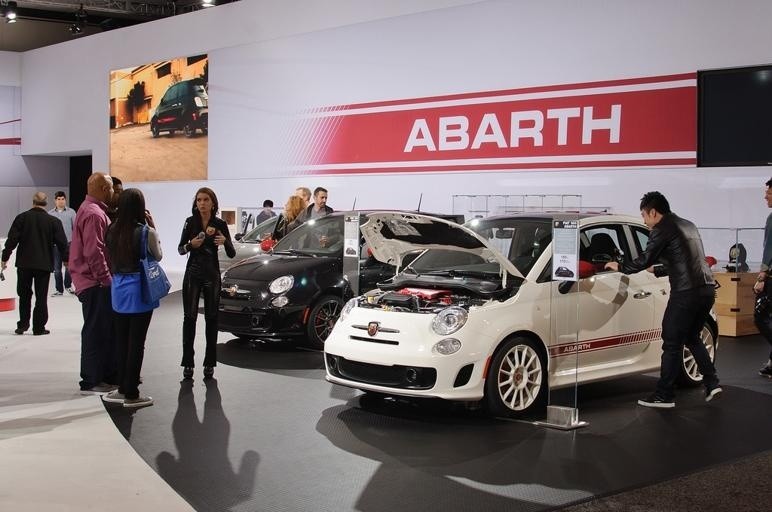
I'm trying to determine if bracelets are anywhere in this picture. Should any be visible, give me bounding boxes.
[183,244,190,258]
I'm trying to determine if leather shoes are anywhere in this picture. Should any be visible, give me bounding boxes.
[183,367,193,377]
[35,330,49,335]
[15,329,22,333]
[203,367,213,376]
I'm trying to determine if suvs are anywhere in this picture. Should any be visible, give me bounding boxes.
[150,78,208,138]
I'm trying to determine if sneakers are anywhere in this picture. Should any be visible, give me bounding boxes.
[705,383,722,404]
[124,397,153,408]
[84,383,119,394]
[52,291,60,296]
[760,365,772,377]
[66,287,73,294]
[104,392,127,404]
[638,395,675,409]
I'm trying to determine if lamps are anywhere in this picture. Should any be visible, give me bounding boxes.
[6,1,18,19]
[6,20,17,24]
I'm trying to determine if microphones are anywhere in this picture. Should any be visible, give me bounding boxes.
[196,233,205,243]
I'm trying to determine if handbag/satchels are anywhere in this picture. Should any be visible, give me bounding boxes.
[143,225,170,303]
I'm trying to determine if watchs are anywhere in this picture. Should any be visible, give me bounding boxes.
[756,276,765,282]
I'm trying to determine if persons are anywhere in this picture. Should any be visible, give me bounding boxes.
[604,191,724,409]
[46,191,76,296]
[67,172,115,391]
[257,199,277,225]
[287,188,334,235]
[177,187,236,378]
[276,194,306,240]
[295,187,314,208]
[102,187,163,408]
[0,190,69,336]
[105,177,123,223]
[752,179,772,376]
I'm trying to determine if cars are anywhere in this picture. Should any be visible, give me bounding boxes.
[215,209,438,349]
[217,213,282,273]
[323,211,719,422]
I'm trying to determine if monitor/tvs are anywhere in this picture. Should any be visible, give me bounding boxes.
[696,63,772,166]
